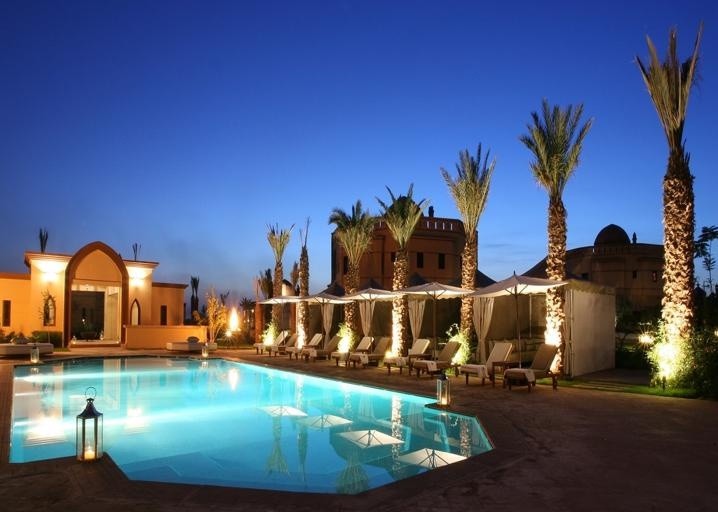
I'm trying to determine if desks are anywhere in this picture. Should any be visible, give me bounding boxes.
[409,354,433,375]
[303,345,318,360]
[349,348,369,354]
[492,361,518,387]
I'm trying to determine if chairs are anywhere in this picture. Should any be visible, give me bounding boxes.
[350,338,392,369]
[265,334,299,357]
[254,331,289,354]
[300,336,343,362]
[413,341,461,378]
[460,343,513,386]
[502,344,560,392]
[384,339,430,373]
[285,333,322,360]
[331,337,374,367]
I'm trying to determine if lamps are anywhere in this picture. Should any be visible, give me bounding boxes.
[75,386,105,463]
[434,371,452,408]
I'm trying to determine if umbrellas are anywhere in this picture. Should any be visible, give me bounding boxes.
[336,396,405,451]
[463,271,570,369]
[336,287,408,353]
[294,290,356,348]
[392,429,468,470]
[295,396,353,432]
[257,381,308,418]
[256,295,301,346]
[392,280,475,361]
